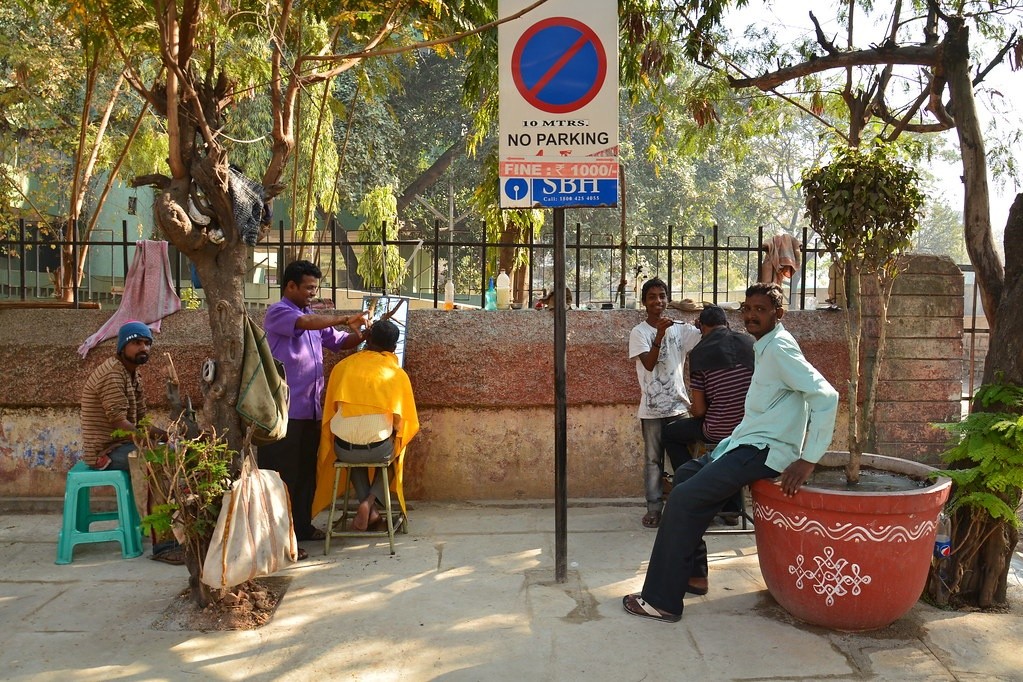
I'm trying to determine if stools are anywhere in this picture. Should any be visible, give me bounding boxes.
[324,459,408,555]
[694,440,746,530]
[55,461,144,565]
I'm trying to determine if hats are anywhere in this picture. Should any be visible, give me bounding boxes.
[117,322,153,354]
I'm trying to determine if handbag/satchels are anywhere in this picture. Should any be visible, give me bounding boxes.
[662,472,674,492]
[200,446,299,589]
[234,314,291,441]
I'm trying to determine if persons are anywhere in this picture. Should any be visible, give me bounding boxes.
[329,319,398,531]
[628,276,758,528]
[81,321,184,564]
[536,286,573,310]
[623,283,840,624]
[257,260,373,561]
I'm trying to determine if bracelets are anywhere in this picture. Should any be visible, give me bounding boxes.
[343,317,349,326]
[652,342,661,348]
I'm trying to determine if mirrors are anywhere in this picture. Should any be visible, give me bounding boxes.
[356,296,409,369]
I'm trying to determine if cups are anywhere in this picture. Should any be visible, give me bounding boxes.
[804,296,818,310]
[601,303,613,308]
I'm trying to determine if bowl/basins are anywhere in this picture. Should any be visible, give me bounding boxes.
[510,304,522,309]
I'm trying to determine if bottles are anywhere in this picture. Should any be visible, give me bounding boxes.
[496,269,510,310]
[485,277,496,311]
[717,302,746,310]
[445,278,454,310]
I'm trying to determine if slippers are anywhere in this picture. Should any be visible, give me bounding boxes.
[686,584,708,594]
[149,548,185,565]
[622,595,681,622]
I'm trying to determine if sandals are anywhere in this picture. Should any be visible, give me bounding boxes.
[310,529,326,540]
[298,549,308,559]
[642,511,661,527]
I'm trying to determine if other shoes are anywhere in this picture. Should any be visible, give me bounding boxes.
[725,516,739,525]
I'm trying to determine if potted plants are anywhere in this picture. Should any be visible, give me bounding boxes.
[747,144,953,633]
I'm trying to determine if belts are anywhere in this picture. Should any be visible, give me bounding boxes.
[334,435,390,449]
